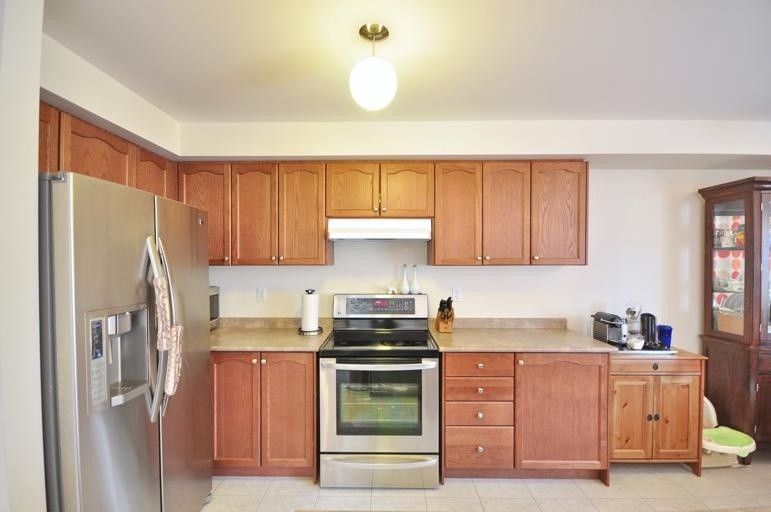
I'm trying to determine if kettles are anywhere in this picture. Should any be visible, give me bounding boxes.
[640,313,656,344]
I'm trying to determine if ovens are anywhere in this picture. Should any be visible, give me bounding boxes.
[319,358,439,489]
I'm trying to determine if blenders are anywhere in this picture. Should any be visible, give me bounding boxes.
[626,308,644,349]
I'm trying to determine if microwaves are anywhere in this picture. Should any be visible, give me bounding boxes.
[210,286,219,330]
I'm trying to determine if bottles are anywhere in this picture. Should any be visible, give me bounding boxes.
[400,264,409,295]
[411,264,420,295]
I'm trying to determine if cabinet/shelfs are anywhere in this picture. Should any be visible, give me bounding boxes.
[435,160,530,265]
[59,109,178,201]
[178,161,231,266]
[231,161,334,265]
[515,352,610,486]
[530,160,588,265]
[441,353,515,484]
[212,351,318,484]
[698,176,771,465]
[326,160,435,219]
[611,349,708,477]
[38,98,58,173]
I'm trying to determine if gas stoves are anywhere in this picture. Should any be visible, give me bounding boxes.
[319,330,439,358]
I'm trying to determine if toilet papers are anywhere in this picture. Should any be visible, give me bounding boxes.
[301,294,320,332]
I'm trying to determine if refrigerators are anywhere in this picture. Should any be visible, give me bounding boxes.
[39,172,212,512]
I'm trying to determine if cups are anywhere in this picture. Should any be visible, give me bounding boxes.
[657,325,672,348]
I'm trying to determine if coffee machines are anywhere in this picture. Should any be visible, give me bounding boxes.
[593,312,627,347]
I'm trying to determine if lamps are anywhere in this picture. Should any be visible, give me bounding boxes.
[349,24,396,111]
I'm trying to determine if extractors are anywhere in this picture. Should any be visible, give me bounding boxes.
[327,219,432,242]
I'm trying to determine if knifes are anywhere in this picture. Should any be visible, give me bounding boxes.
[435,297,454,333]
